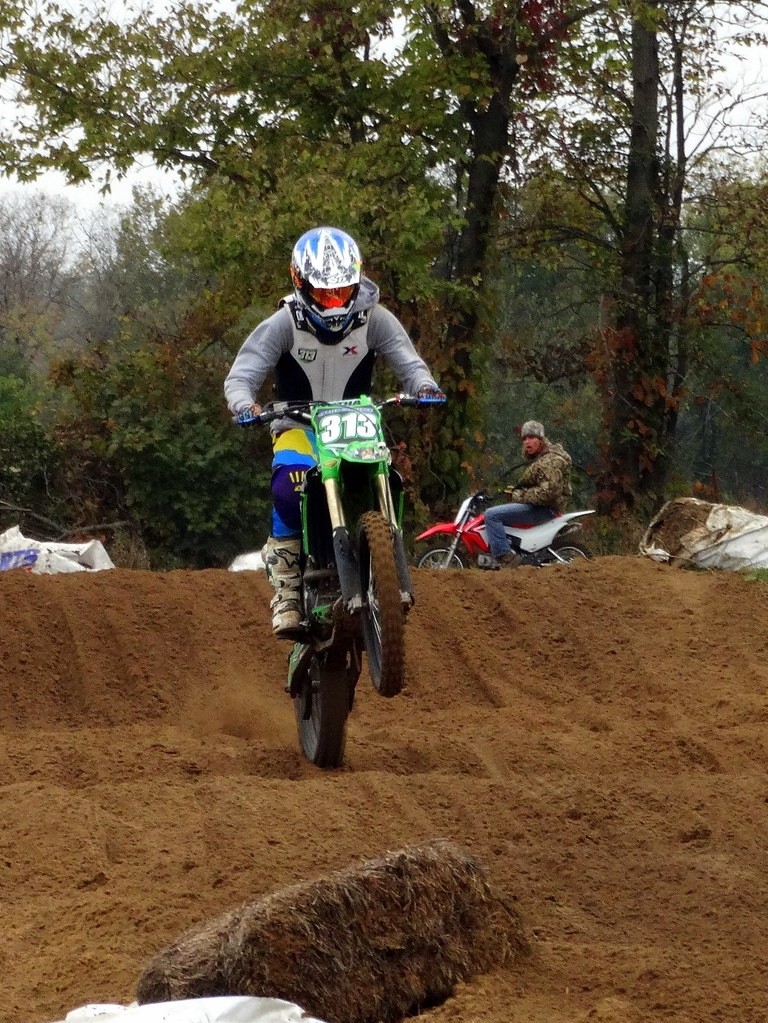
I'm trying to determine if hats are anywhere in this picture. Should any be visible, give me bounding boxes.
[521,420,545,440]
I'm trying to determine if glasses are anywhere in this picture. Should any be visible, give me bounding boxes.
[303,284,356,308]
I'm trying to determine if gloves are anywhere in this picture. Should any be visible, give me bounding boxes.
[237,403,262,429]
[503,485,514,501]
[416,387,446,411]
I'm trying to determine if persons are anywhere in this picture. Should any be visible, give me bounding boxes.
[222,225,445,637]
[483,420,572,568]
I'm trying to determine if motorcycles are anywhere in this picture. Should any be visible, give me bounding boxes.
[413,474,600,571]
[227,388,448,770]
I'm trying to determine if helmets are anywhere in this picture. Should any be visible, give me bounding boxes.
[290,227,361,333]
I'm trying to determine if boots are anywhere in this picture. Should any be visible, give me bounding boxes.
[266,532,305,638]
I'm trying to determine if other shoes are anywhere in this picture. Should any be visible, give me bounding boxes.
[496,551,522,569]
